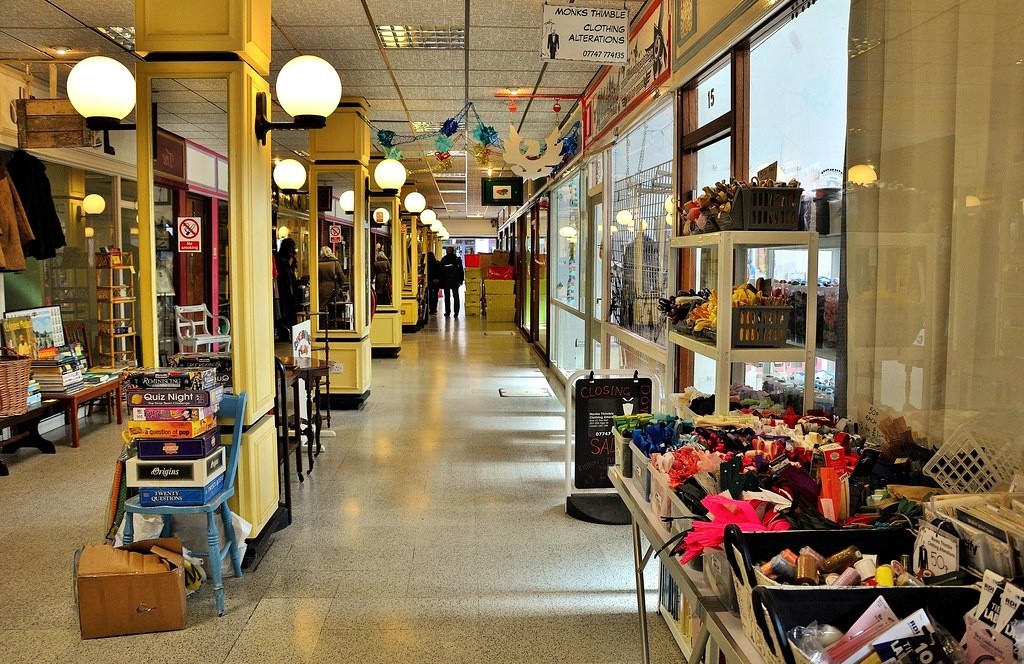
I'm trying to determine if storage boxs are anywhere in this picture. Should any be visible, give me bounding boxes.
[462,249,516,324]
[73,535,190,640]
[614,420,1024,664]
[124,353,231,505]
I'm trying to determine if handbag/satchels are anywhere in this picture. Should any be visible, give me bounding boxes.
[329,291,346,313]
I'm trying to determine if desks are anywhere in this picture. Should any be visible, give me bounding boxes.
[272,354,339,474]
[607,463,783,662]
[0,400,68,476]
[35,373,126,448]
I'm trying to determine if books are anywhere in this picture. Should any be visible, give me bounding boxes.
[1,316,109,405]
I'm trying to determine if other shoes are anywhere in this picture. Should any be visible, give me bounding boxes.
[429,309,437,314]
[444,312,450,316]
[454,313,458,317]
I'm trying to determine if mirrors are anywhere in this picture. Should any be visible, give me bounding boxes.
[369,202,392,305]
[150,76,231,392]
[401,219,436,293]
[315,171,353,330]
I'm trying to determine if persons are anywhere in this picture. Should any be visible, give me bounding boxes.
[441,247,464,316]
[19,335,30,356]
[277,238,308,330]
[197,372,203,380]
[428,253,444,314]
[182,411,191,421]
[372,251,391,305]
[318,245,345,329]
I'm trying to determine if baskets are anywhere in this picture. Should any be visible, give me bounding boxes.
[923,423,1022,495]
[700,306,794,346]
[713,186,804,231]
[0,346,31,417]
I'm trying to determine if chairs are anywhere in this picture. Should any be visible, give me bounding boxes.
[273,356,306,494]
[173,302,233,352]
[123,392,249,618]
[65,322,114,423]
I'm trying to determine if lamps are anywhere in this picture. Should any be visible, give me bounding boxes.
[74,193,110,222]
[364,159,406,199]
[273,158,310,196]
[371,207,389,228]
[399,191,449,241]
[254,54,342,144]
[558,192,675,238]
[69,54,140,134]
[338,190,355,215]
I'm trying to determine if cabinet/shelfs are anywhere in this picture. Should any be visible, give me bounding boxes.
[96,250,137,416]
[665,230,829,415]
